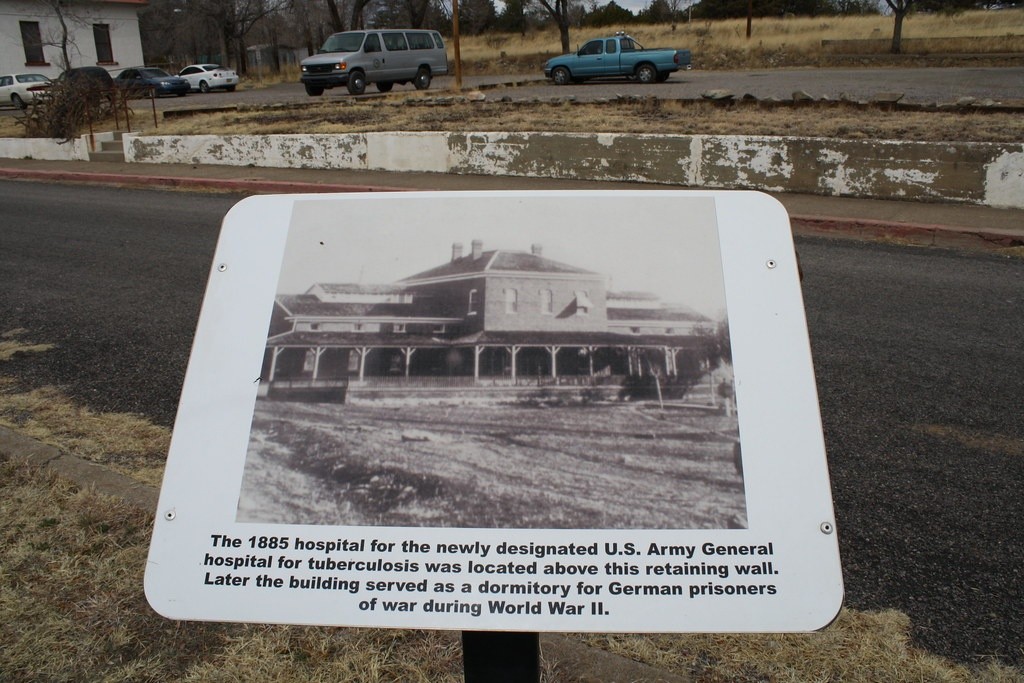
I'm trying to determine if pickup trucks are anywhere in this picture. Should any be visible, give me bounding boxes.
[543,31,691,84]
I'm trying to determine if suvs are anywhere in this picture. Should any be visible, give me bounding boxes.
[55,67,116,101]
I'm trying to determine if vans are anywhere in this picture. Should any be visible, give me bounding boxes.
[298,30,449,95]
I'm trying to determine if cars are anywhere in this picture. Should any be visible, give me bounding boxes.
[0,73,54,110]
[173,64,239,93]
[114,68,191,98]
